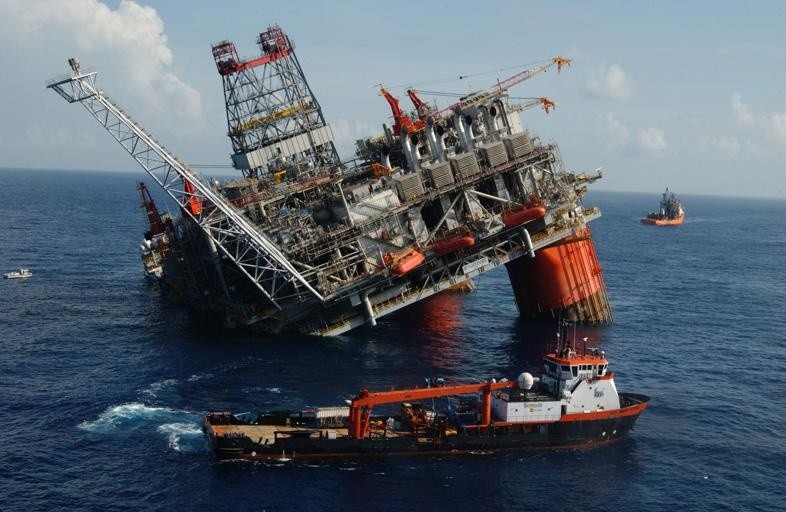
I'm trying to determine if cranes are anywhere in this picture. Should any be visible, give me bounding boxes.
[373,57,572,136]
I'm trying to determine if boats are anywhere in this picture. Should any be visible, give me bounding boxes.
[3,269,32,278]
[640,187,684,225]
[201,311,651,461]
